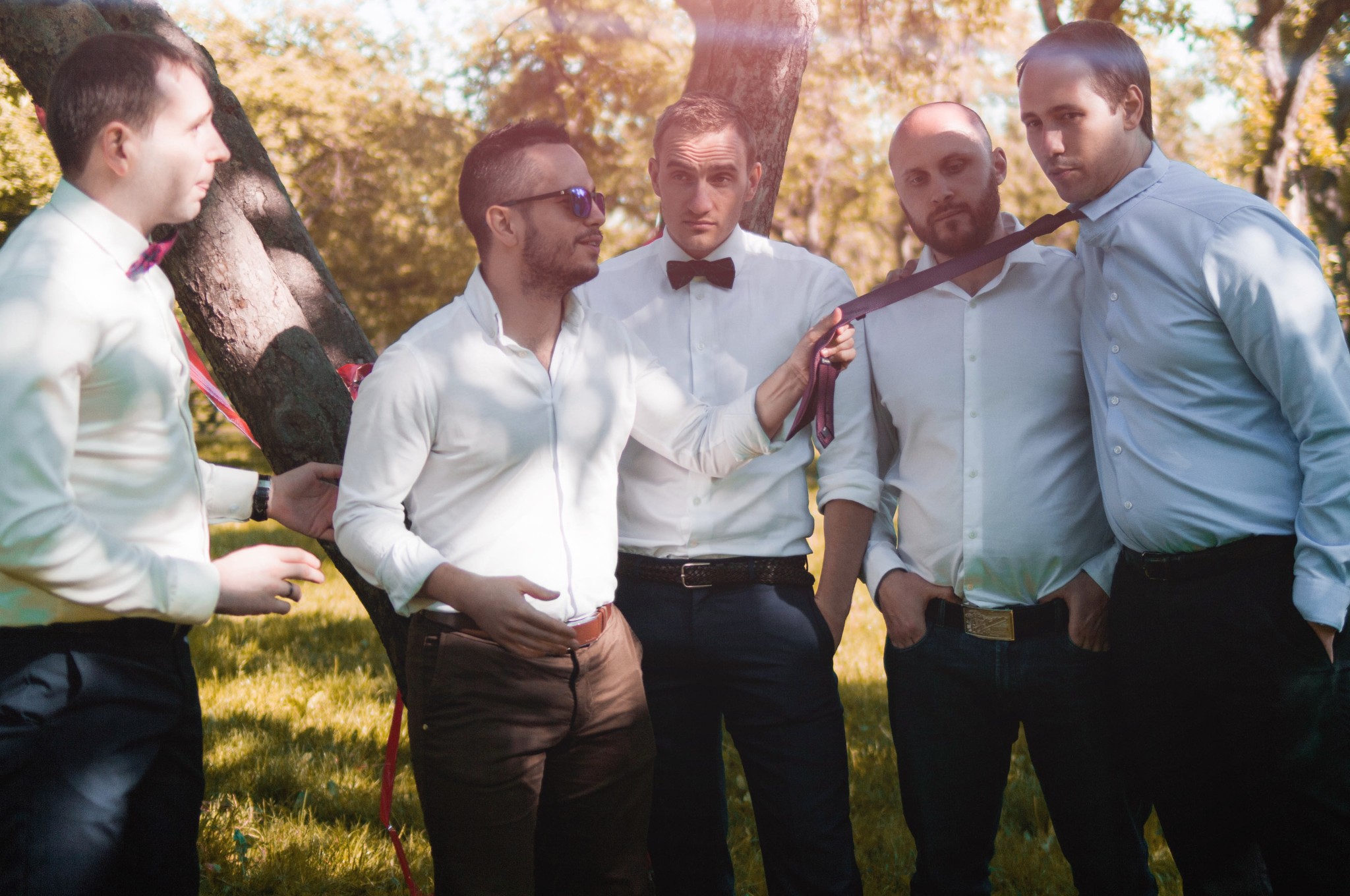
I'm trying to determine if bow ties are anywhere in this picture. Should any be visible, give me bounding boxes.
[667,257,735,290]
[126,228,178,281]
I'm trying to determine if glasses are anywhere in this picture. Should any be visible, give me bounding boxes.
[499,187,606,219]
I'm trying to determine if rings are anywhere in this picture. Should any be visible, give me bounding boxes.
[280,581,293,597]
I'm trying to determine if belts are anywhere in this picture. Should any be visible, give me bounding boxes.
[417,602,612,648]
[1123,536,1294,583]
[926,598,1069,642]
[616,558,815,587]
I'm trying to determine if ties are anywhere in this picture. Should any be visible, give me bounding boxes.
[786,208,1088,449]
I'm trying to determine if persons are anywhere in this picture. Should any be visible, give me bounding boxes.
[0,29,348,896]
[335,21,1350,896]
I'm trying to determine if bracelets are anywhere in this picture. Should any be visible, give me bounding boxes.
[250,473,272,523]
